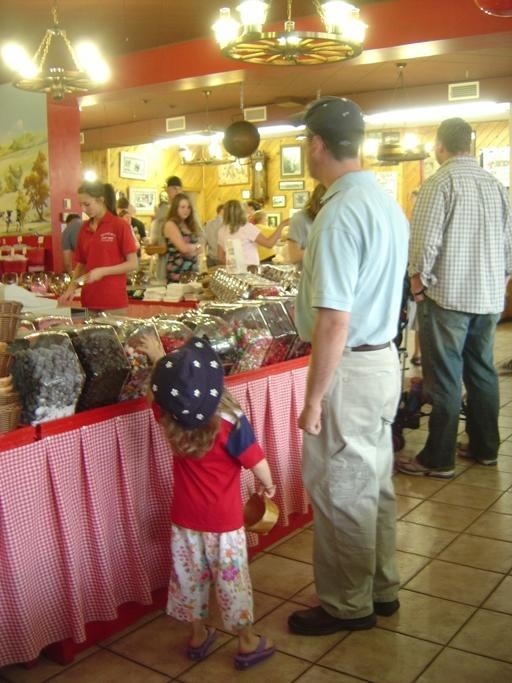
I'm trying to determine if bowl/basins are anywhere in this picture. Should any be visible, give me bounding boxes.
[243,490,279,536]
[145,244,167,256]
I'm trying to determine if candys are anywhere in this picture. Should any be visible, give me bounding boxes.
[119,302,312,400]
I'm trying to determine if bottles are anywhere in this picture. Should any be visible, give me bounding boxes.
[2,270,71,296]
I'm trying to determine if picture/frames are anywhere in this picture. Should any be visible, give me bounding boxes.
[279,180,304,190]
[292,191,310,209]
[242,188,250,199]
[271,195,286,208]
[127,187,157,217]
[362,130,509,205]
[265,213,281,229]
[289,208,302,218]
[216,161,250,185]
[120,150,147,182]
[280,144,304,177]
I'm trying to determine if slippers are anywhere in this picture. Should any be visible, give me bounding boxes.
[235,633,276,669]
[186,627,216,664]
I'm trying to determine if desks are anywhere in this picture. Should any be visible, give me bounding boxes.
[1,351,316,674]
[42,281,197,317]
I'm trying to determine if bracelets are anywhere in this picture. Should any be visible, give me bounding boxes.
[412,288,424,296]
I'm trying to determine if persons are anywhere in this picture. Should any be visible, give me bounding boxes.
[134,330,279,671]
[409,331,422,367]
[54,176,290,314]
[287,184,327,269]
[394,116,511,481]
[285,94,411,637]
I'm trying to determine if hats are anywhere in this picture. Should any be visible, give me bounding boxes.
[152,337,223,427]
[288,97,365,144]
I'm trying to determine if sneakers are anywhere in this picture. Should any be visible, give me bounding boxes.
[291,598,398,636]
[397,456,457,478]
[457,440,497,466]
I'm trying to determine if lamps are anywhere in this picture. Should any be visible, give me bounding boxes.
[2,1,116,101]
[212,1,367,67]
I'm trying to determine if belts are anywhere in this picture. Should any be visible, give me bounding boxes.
[351,340,393,355]
